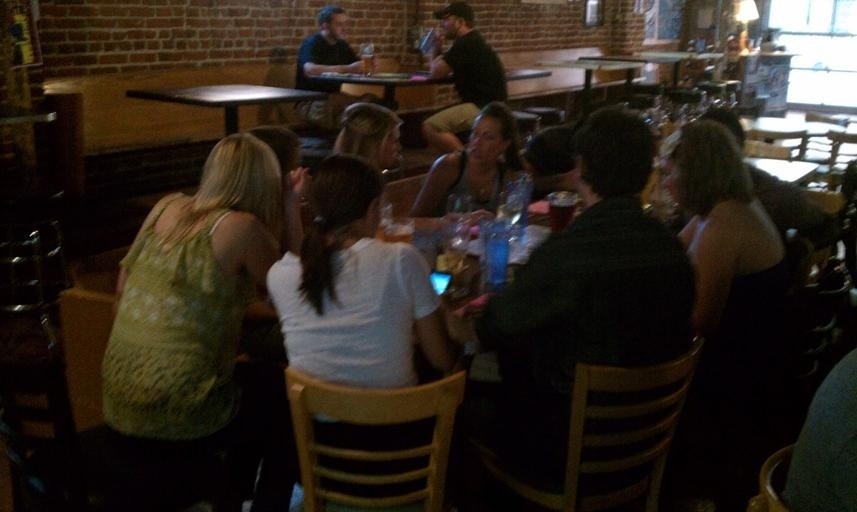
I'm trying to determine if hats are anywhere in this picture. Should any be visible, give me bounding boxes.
[433,1,473,22]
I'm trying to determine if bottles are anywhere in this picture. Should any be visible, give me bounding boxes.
[485,221,510,287]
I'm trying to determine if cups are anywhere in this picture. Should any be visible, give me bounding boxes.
[420,27,435,55]
[375,203,416,244]
[547,191,579,232]
[361,53,375,77]
[447,193,473,221]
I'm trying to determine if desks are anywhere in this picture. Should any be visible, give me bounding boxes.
[124,49,743,138]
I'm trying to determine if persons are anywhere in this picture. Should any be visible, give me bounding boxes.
[465,105,698,509]
[100,132,309,512]
[419,1,508,154]
[332,98,402,181]
[697,108,840,272]
[659,120,798,410]
[410,98,523,217]
[524,122,586,206]
[264,154,474,508]
[293,4,375,125]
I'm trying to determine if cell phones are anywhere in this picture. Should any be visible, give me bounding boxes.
[428,266,454,302]
